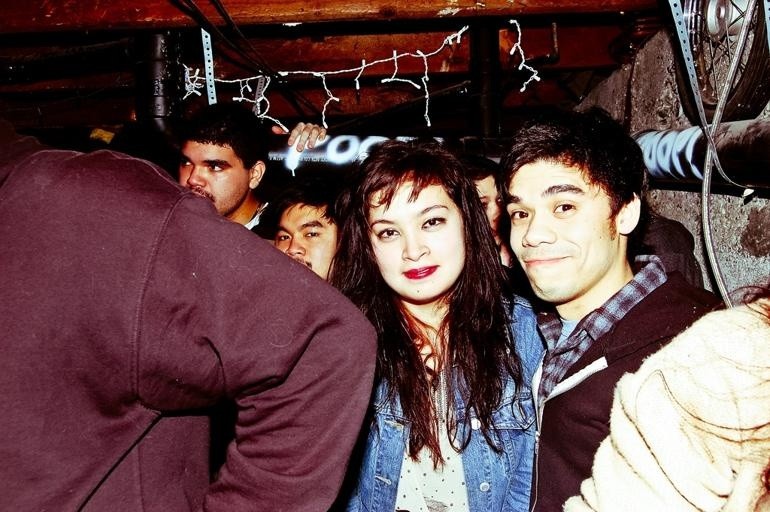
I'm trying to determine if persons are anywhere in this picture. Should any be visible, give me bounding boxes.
[504,114,727,512]
[175,100,274,240]
[270,121,328,153]
[558,284,770,512]
[0,114,379,512]
[465,165,512,269]
[274,184,343,280]
[331,135,547,512]
